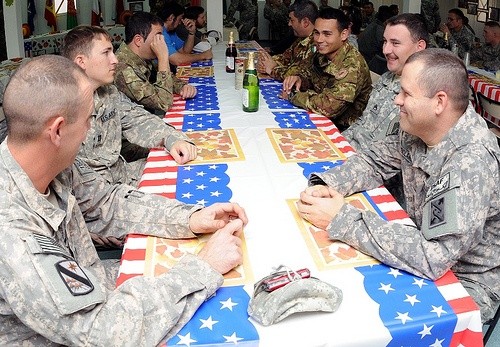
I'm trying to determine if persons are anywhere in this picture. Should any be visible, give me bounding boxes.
[111,12,198,114]
[338,0,402,74]
[281,8,374,132]
[256,0,319,83]
[296,49,500,332]
[64,23,197,248]
[415,7,500,76]
[159,5,214,64]
[421,0,441,36]
[319,0,333,9]
[0,54,247,347]
[345,14,429,207]
[177,7,216,52]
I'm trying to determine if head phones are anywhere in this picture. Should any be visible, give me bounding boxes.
[207,30,220,42]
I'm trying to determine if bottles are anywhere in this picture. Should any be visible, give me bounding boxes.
[442,31,451,51]
[242,51,259,112]
[225,31,237,73]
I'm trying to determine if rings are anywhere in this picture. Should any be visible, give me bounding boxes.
[306,214,308,220]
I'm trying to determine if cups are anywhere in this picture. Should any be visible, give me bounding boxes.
[451,43,458,55]
[235,56,246,90]
[463,52,470,66]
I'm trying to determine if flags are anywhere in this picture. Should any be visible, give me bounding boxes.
[66,0,77,30]
[27,0,36,34]
[112,0,123,25]
[91,0,104,27]
[43,0,58,33]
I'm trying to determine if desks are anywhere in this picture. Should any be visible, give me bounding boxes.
[115,41,500,347]
[25,29,70,57]
[0,55,31,102]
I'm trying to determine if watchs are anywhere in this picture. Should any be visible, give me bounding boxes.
[288,91,295,101]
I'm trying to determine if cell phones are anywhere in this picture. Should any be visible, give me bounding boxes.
[262,268,311,291]
